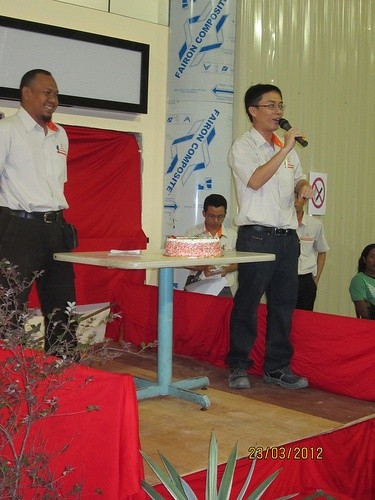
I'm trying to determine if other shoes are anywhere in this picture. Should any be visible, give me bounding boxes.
[263,367,308,389]
[228,368,250,389]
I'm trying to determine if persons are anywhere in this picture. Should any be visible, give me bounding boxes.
[0,69,81,363]
[224,84,309,390]
[349,244,375,320]
[294,193,330,311]
[185,194,238,297]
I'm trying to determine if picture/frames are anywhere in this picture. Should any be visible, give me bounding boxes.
[0,16,150,114]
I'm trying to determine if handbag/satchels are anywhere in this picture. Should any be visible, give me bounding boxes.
[61,223,79,249]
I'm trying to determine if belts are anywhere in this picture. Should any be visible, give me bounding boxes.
[4,207,63,224]
[239,225,297,237]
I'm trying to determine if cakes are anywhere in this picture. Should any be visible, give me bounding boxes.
[165,235,222,257]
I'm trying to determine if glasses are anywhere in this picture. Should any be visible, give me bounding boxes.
[205,213,224,220]
[252,103,287,110]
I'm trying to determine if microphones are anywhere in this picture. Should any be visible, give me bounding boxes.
[278,118,308,148]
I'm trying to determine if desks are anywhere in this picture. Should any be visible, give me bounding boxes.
[53,250,275,411]
[0,339,147,500]
